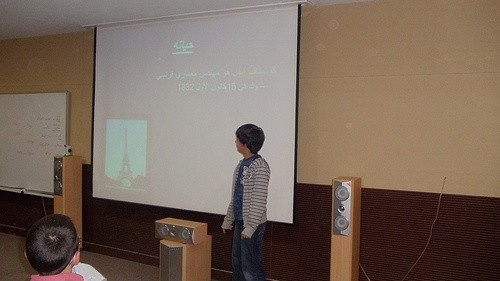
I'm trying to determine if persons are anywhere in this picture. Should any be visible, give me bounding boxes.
[24,214,84,281]
[222,124,271,281]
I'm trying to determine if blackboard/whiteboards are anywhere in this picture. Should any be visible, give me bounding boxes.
[0,91,70,198]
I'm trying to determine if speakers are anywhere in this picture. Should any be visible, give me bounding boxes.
[329,177,362,281]
[55,156,82,248]
[155,218,207,245]
